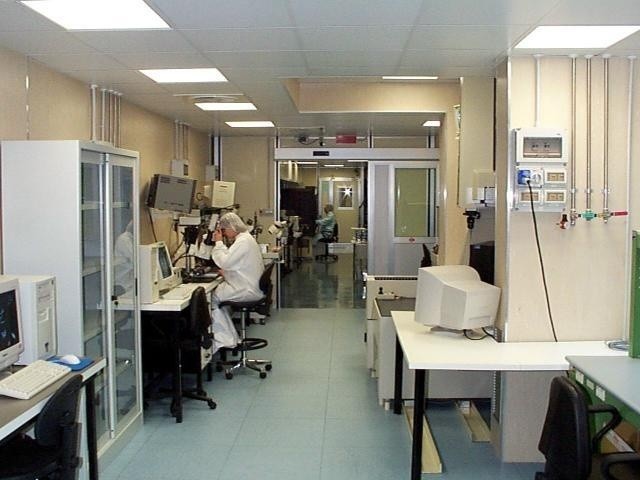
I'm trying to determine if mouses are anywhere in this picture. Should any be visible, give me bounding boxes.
[63,355,80,364]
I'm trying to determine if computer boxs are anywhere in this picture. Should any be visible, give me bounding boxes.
[7,275,55,365]
[139,244,159,304]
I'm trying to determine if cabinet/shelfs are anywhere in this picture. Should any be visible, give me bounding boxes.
[1,137,146,470]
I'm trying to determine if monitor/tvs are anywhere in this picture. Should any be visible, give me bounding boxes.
[414,264,501,334]
[0,274,24,371]
[150,241,176,290]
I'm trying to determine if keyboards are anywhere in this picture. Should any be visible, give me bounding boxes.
[165,284,197,300]
[0,360,71,400]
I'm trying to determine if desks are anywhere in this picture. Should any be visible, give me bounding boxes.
[140,272,224,425]
[564,352,640,452]
[389,310,630,480]
[95,290,137,421]
[1,353,108,480]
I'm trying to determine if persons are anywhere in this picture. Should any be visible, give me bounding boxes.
[313,203,336,246]
[210,212,267,356]
[115,215,134,304]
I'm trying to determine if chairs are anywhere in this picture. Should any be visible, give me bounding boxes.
[98,282,137,415]
[216,260,277,378]
[154,285,217,422]
[314,223,339,261]
[534,375,640,477]
[2,372,85,480]
